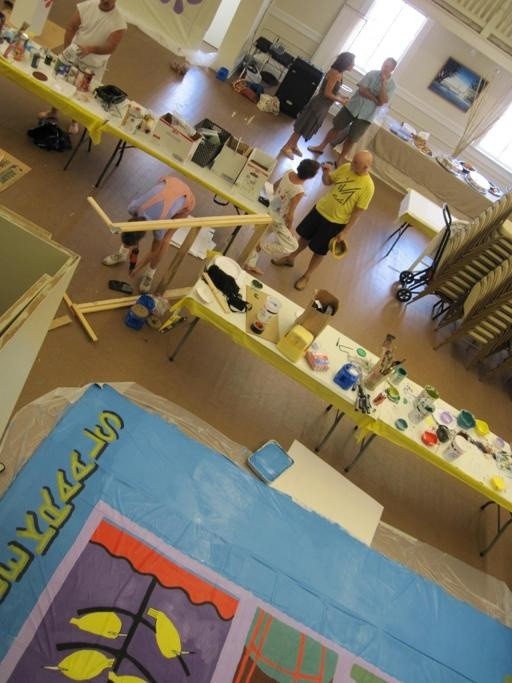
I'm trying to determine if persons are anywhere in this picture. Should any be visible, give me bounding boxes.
[280,52,356,159]
[101,175,197,294]
[243,159,322,276]
[308,56,398,168]
[38,0,128,135]
[268,149,377,291]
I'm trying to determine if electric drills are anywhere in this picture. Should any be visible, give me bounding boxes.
[129,247,139,271]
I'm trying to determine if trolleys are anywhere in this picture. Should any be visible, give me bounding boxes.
[396,203,457,319]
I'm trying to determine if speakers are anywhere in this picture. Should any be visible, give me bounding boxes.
[273,55,323,120]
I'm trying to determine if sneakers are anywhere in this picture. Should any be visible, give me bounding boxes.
[295,276,308,291]
[139,269,155,293]
[271,256,294,267]
[281,145,302,160]
[307,145,324,153]
[334,161,338,169]
[102,252,128,266]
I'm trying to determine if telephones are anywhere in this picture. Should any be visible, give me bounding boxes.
[269,36,288,56]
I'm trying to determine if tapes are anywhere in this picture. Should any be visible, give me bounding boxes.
[146,315,162,329]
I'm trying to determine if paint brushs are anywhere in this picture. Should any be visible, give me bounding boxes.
[364,334,406,391]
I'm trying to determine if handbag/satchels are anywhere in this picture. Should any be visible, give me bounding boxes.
[233,64,280,116]
[201,265,253,313]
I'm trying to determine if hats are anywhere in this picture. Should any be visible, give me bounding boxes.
[328,236,349,259]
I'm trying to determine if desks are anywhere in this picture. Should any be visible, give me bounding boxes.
[0,2,512,682]
